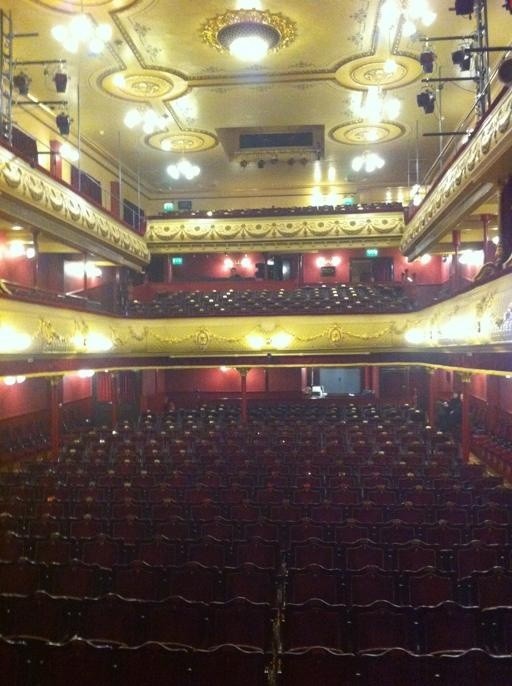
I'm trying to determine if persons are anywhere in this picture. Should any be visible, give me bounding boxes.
[431,391,464,434]
[229,266,242,279]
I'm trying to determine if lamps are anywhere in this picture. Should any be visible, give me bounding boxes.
[224,256,251,274]
[312,155,339,208]
[402,250,486,272]
[316,256,341,275]
[64,259,102,280]
[49,0,201,182]
[218,23,280,65]
[349,0,437,174]
